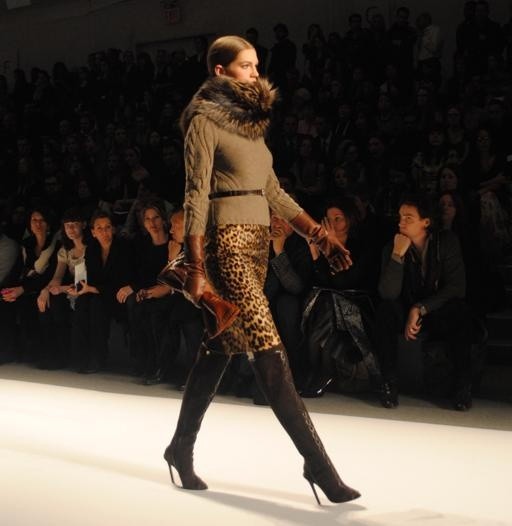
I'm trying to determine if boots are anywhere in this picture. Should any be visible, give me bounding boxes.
[164,342,231,490]
[247,350,361,505]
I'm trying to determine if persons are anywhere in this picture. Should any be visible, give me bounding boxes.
[162,35,363,505]
[2,34,246,398]
[245,2,510,410]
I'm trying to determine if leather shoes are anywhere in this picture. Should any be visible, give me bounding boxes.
[373,380,399,408]
[299,372,336,398]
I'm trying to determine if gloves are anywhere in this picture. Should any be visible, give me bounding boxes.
[183,235,214,309]
[287,209,353,272]
